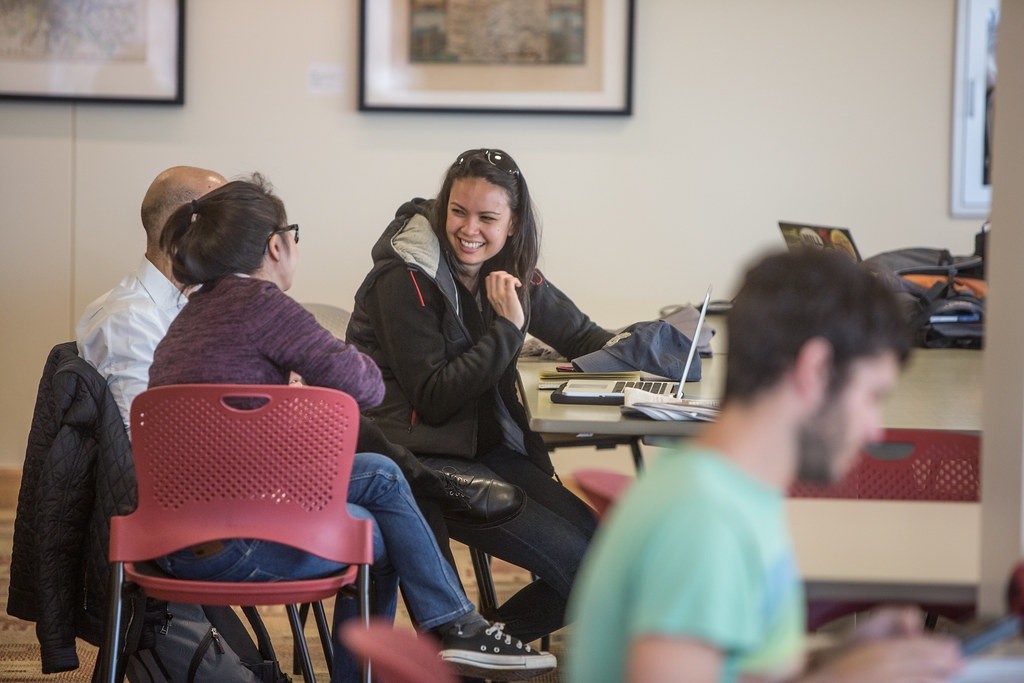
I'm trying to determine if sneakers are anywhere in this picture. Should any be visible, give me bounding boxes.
[437,622,557,680]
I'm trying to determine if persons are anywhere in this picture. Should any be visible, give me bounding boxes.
[143,170,558,683]
[75,166,228,441]
[343,147,619,683]
[562,246,965,682]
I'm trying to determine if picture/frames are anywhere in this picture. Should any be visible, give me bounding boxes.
[0,0,187,108]
[357,0,636,116]
[949,0,999,220]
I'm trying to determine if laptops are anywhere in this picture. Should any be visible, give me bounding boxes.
[563,285,714,400]
[780,223,864,265]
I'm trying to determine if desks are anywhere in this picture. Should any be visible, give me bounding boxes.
[785,496,982,635]
[702,314,729,356]
[515,349,981,478]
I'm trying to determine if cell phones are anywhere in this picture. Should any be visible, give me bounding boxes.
[961,616,1021,656]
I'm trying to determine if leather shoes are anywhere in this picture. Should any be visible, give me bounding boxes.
[430,466,527,529]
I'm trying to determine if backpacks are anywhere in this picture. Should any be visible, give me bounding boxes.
[124,600,292,683]
[863,247,985,326]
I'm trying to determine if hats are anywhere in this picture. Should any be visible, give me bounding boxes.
[570,320,702,383]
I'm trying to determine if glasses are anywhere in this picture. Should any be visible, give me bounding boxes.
[456,148,520,182]
[262,223,300,254]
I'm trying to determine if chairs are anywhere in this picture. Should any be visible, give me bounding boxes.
[788,427,981,503]
[107,384,375,683]
[7,341,334,683]
